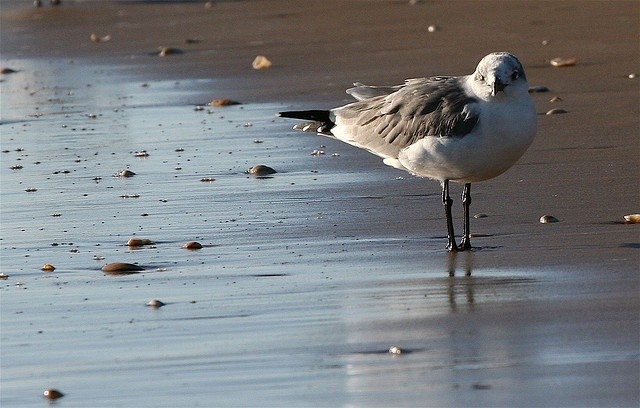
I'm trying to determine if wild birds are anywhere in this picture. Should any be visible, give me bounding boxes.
[275,50,541,254]
